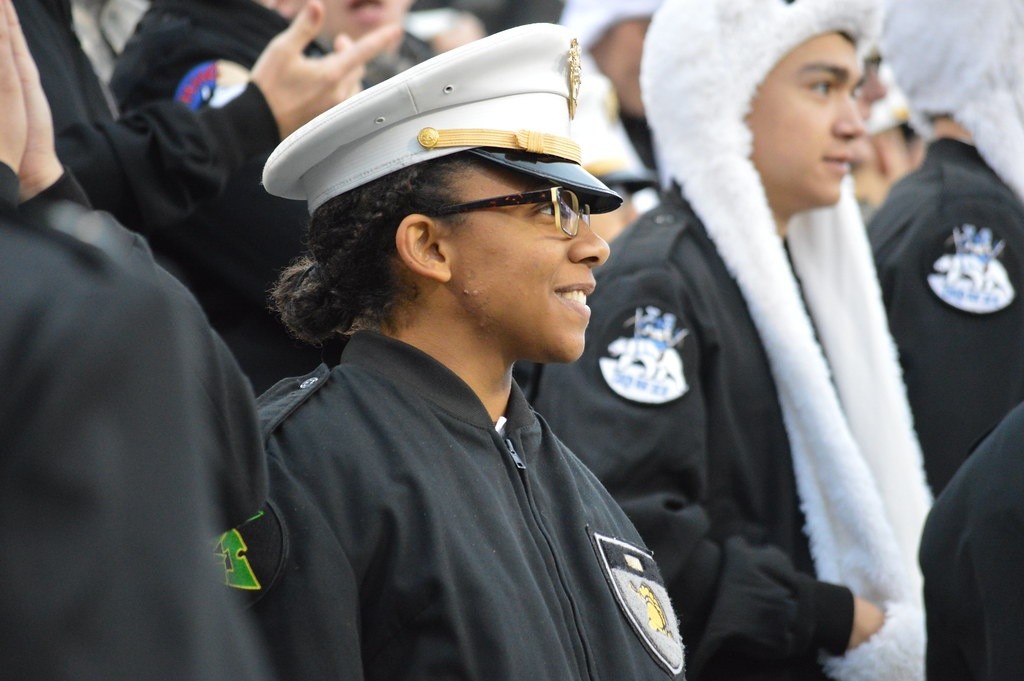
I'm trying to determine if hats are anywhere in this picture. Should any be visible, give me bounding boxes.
[557,0,658,51]
[638,0,882,200]
[260,20,624,217]
[859,58,912,136]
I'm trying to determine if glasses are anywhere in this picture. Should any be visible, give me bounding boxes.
[420,185,593,238]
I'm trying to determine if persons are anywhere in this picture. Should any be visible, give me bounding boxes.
[0,0,1023,681]
[258,23,686,681]
